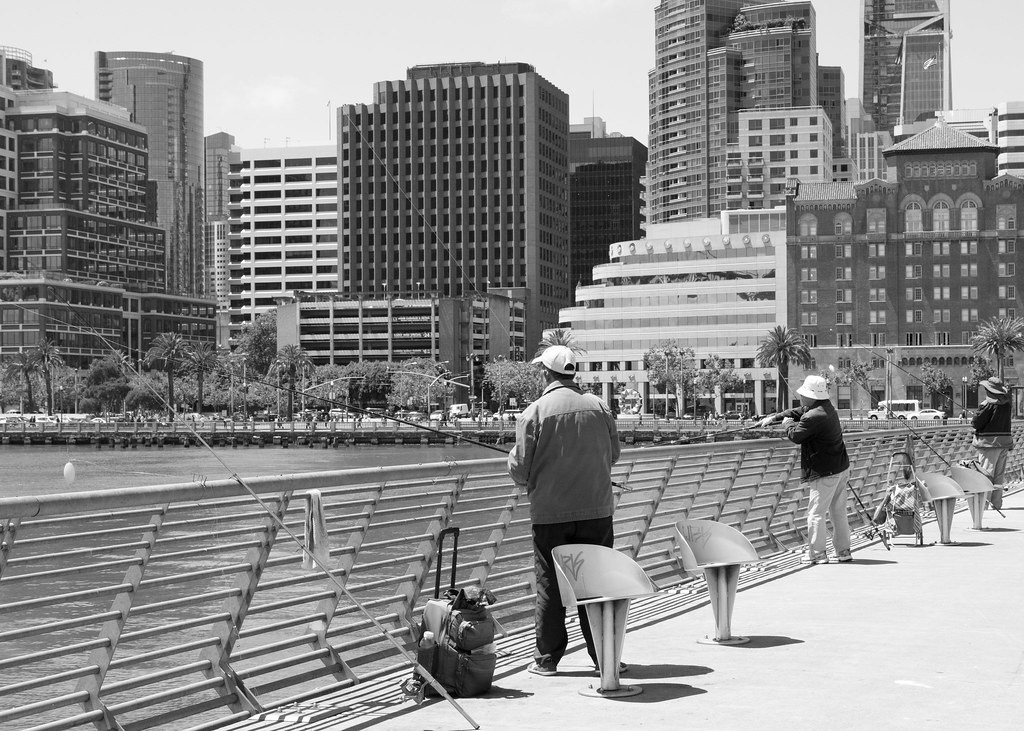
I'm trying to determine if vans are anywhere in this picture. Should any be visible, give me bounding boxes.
[867,399,919,421]
[492,410,524,422]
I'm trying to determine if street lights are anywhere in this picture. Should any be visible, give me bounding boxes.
[427,371,452,421]
[400,362,417,419]
[465,352,478,422]
[962,376,968,419]
[493,354,508,421]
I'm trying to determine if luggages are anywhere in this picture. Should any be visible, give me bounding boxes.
[885,452,923,548]
[403,527,507,698]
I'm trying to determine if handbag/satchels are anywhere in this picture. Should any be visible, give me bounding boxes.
[873,493,890,524]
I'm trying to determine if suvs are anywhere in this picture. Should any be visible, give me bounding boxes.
[328,409,356,420]
[719,410,743,420]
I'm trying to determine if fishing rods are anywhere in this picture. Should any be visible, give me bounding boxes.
[785,321,1007,521]
[14,245,483,730]
[8,300,633,494]
[769,359,896,563]
[840,327,977,417]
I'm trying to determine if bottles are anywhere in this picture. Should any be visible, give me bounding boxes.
[418,631,435,647]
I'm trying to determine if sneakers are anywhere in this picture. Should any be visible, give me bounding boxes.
[828,549,852,560]
[800,552,829,563]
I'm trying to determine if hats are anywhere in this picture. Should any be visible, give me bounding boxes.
[532,345,577,375]
[797,375,829,400]
[979,376,1008,394]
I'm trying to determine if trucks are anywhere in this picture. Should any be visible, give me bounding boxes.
[450,404,468,419]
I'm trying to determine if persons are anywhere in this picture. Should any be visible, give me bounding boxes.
[477,412,516,428]
[971,376,1013,510]
[27,415,36,429]
[440,410,458,428]
[701,410,752,429]
[506,344,630,676]
[754,375,853,564]
[770,406,776,414]
[275,413,366,432]
[55,415,60,428]
[637,412,643,427]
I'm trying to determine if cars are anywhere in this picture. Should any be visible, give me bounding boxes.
[394,410,427,422]
[756,413,777,421]
[0,410,202,423]
[469,408,493,417]
[430,410,449,421]
[906,408,946,421]
[683,413,697,420]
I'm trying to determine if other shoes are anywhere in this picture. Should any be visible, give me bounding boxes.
[527,660,557,675]
[594,659,627,674]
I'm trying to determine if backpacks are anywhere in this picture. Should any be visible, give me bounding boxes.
[960,459,994,486]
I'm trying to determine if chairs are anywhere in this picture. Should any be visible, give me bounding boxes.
[675,520,763,644]
[915,470,966,546]
[551,542,657,698]
[950,463,1002,533]
